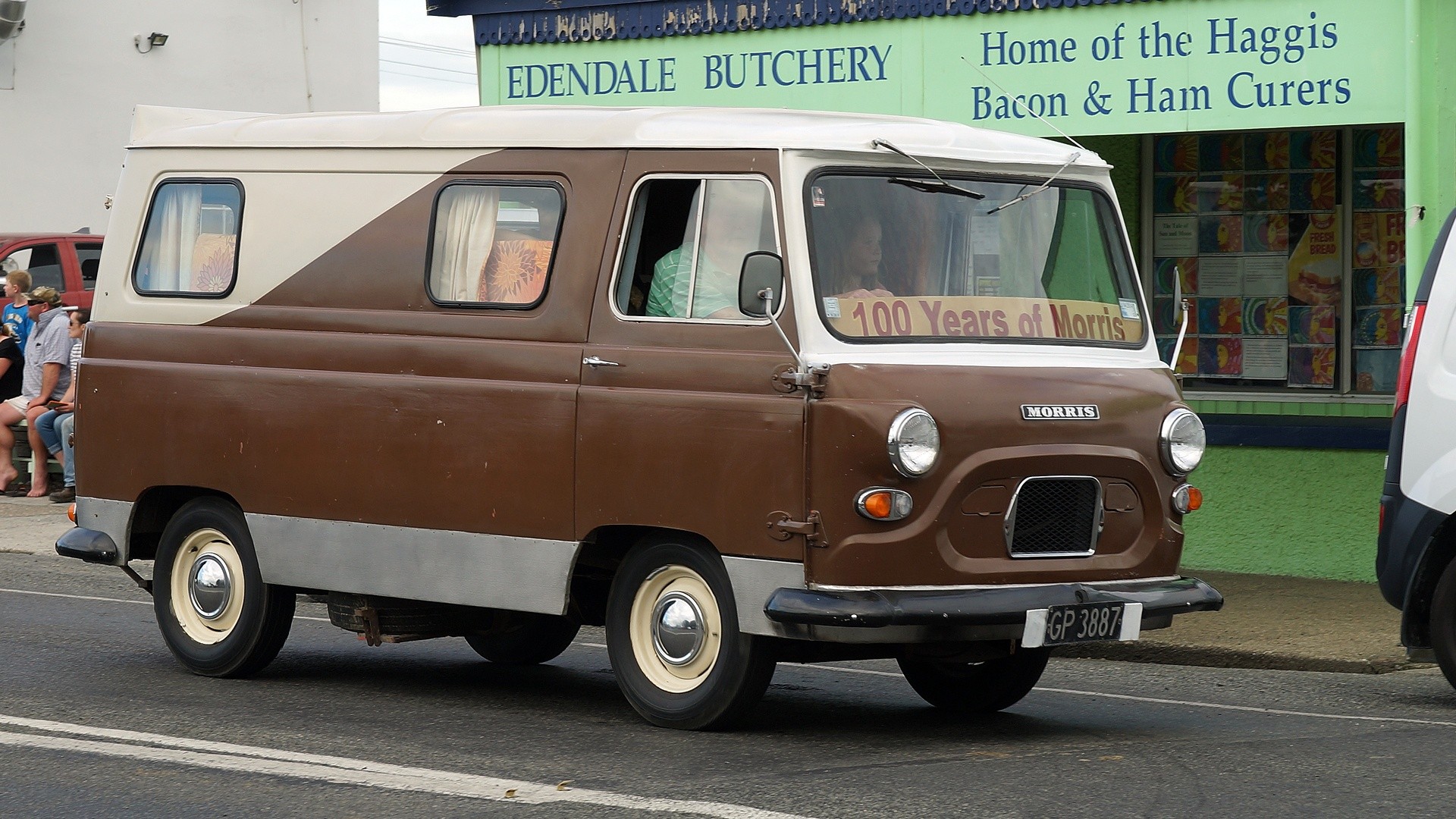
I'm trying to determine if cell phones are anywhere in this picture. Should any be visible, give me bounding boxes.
[47,401,68,408]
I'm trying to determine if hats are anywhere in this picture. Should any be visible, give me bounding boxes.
[21,286,62,307]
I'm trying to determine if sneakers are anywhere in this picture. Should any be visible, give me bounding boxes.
[49,487,76,501]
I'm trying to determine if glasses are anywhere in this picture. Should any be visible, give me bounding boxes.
[69,321,81,327]
[28,300,46,306]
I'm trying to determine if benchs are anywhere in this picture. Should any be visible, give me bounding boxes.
[10,418,60,488]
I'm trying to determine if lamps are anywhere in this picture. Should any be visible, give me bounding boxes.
[147,31,168,47]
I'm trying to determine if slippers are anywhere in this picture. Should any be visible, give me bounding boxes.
[0,484,31,497]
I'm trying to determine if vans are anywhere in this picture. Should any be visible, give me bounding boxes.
[1375,206,1455,691]
[56,102,1226,734]
[0,226,105,324]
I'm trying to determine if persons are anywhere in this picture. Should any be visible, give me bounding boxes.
[822,202,895,299]
[0,269,81,497]
[49,414,75,503]
[645,204,756,323]
[34,309,91,467]
